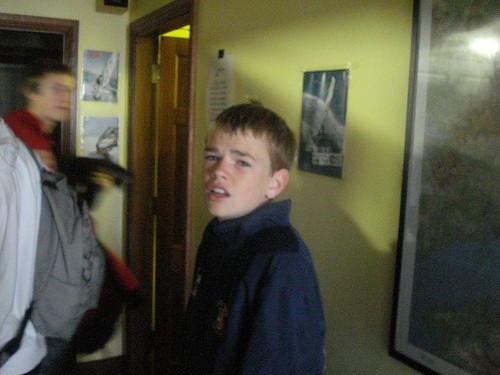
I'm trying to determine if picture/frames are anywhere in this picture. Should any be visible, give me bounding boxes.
[79,113,122,166]
[81,47,124,103]
[294,64,352,181]
[387,2,499,373]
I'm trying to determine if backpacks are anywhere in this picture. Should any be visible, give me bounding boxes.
[26,145,105,338]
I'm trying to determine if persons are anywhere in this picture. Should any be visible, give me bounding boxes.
[0,112,106,375]
[12,61,138,374]
[162,99,326,375]
